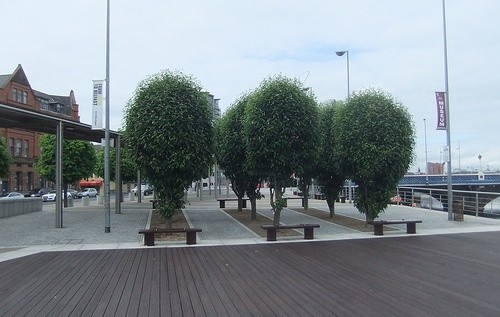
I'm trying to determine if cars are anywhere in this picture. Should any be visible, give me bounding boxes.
[74,188,98,198]
[42,190,78,201]
[0,191,24,197]
[131,185,153,197]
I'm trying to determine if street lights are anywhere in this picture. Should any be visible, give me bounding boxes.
[478,154,482,173]
[424,118,428,176]
[335,51,350,204]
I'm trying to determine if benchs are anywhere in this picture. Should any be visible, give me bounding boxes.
[336,196,347,203]
[138,228,202,246]
[261,223,320,240]
[281,196,310,207]
[293,188,303,196]
[313,194,326,200]
[150,199,186,209]
[216,198,250,208]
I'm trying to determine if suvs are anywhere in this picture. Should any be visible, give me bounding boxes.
[24,187,53,196]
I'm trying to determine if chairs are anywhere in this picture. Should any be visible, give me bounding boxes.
[368,219,422,235]
